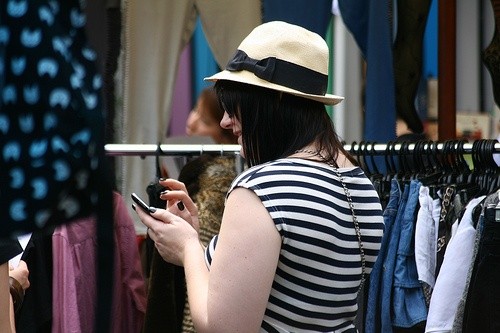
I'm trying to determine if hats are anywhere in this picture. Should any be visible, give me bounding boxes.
[203,21,345,106]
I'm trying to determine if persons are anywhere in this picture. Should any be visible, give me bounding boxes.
[0,222,29,333]
[132,21,385,333]
[142,87,239,333]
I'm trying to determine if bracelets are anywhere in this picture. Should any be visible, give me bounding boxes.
[8,276,24,304]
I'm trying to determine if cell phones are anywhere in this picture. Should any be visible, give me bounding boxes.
[131,193,151,214]
